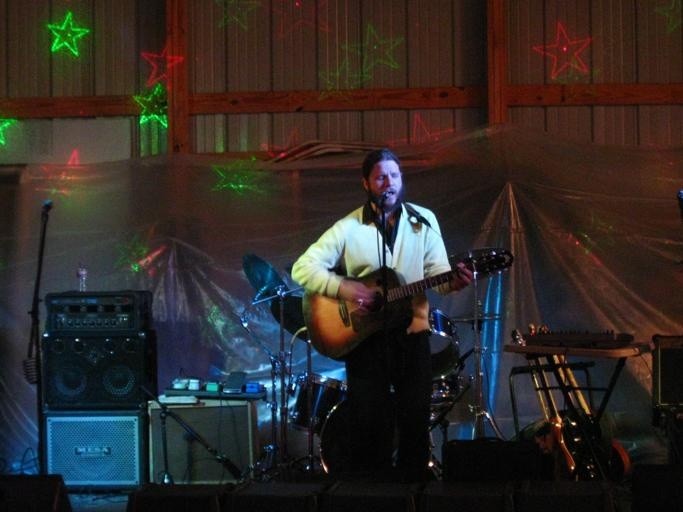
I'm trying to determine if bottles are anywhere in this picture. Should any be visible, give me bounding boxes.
[77,261,87,292]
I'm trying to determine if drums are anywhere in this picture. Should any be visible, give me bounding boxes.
[431,372,460,406]
[320,398,436,483]
[289,373,347,432]
[426,308,459,379]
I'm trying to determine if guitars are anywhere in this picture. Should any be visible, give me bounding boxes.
[302,249,512,361]
[512,323,632,484]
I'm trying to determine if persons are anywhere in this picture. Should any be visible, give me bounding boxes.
[290,147,472,483]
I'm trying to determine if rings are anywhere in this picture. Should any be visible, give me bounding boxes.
[356,298,364,306]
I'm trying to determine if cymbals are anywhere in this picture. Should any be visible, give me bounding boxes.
[242,252,293,301]
[272,297,309,342]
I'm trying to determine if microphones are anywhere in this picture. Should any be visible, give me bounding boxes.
[382,191,391,200]
[40,199,53,241]
[215,455,242,480]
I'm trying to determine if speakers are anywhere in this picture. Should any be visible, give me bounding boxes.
[40,289,158,494]
